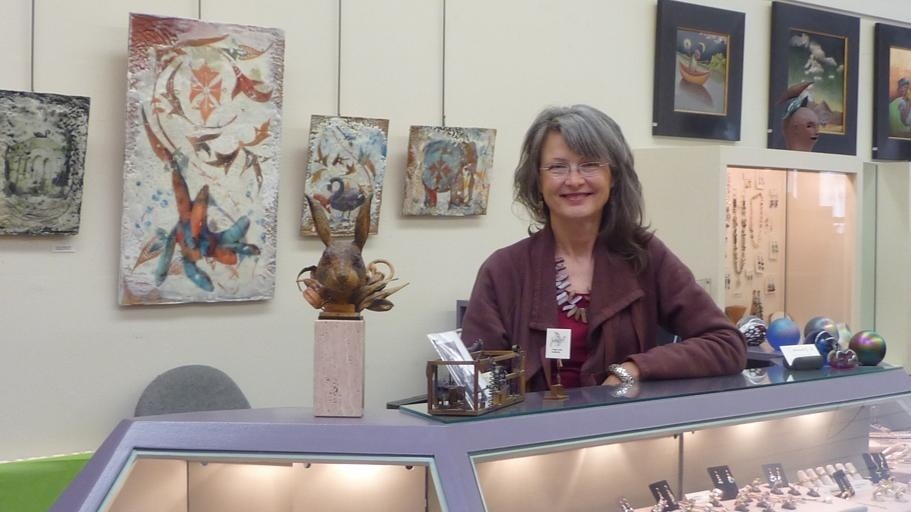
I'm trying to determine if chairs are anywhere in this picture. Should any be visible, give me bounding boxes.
[135,364,251,414]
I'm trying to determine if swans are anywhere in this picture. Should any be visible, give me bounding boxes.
[325,176,366,219]
[32,128,52,138]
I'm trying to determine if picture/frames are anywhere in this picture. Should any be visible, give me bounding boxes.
[651,1,747,142]
[767,1,860,156]
[872,23,908,163]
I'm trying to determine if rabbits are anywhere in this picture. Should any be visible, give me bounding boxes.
[303,191,374,307]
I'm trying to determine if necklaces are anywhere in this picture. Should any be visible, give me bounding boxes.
[553,255,595,326]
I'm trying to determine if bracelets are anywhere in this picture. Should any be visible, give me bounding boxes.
[606,362,634,386]
[607,382,633,397]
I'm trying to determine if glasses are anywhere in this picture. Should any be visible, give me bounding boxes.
[535,158,609,181]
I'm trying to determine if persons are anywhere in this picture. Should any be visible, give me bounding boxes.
[781,105,822,152]
[458,103,751,400]
